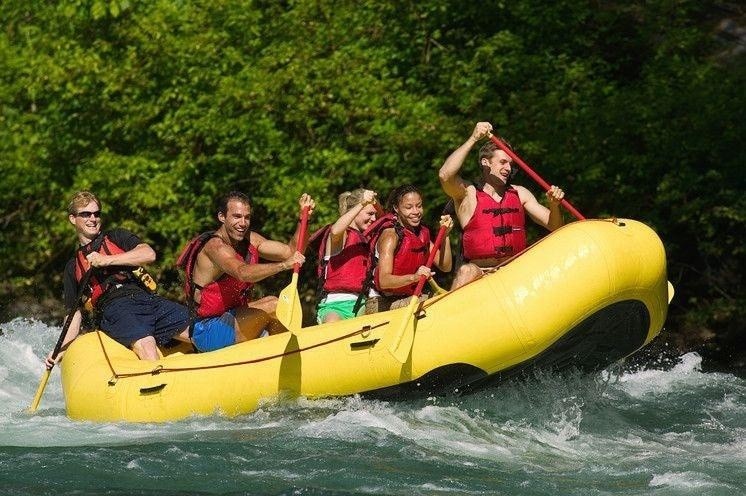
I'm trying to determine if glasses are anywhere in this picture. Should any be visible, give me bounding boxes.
[73,210,102,219]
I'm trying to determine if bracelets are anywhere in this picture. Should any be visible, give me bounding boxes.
[278,263,287,272]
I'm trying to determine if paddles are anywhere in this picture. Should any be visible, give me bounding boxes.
[484,126,676,308]
[373,197,447,296]
[383,218,449,363]
[276,204,310,336]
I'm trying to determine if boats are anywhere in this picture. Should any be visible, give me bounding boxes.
[61,217,673,424]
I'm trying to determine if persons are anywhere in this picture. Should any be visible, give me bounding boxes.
[317,188,385,324]
[45,191,193,370]
[439,122,564,291]
[366,185,455,315]
[188,190,316,352]
